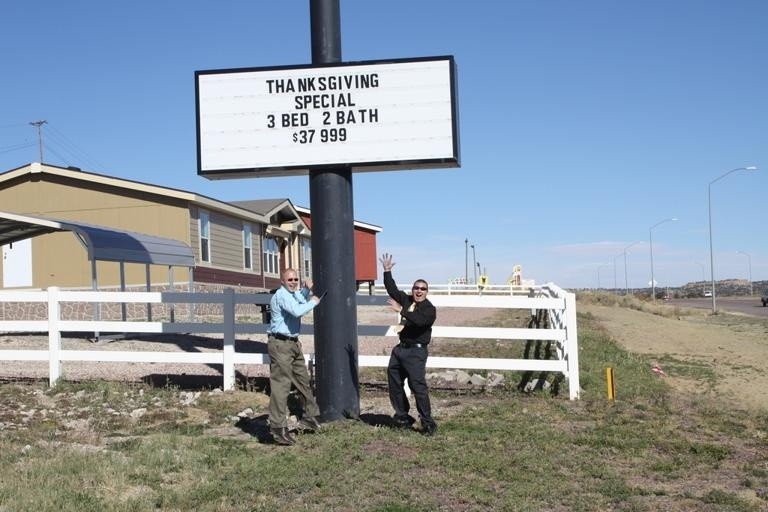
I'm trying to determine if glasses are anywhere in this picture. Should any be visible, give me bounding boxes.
[413,285,427,291]
[287,278,298,281]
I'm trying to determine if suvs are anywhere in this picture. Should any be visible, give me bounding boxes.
[702,290,712,297]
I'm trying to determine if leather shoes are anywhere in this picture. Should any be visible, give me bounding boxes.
[422,422,436,436]
[395,415,408,430]
[302,416,324,431]
[270,426,296,446]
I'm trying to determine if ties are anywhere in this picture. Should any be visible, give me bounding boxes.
[395,302,416,334]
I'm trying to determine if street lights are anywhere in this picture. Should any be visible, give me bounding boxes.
[734,249,754,297]
[611,217,679,302]
[707,165,758,315]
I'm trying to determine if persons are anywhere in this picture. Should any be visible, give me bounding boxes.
[380,252,438,438]
[266,270,322,445]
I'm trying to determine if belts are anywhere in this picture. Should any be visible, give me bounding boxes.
[268,332,297,342]
[400,342,427,349]
